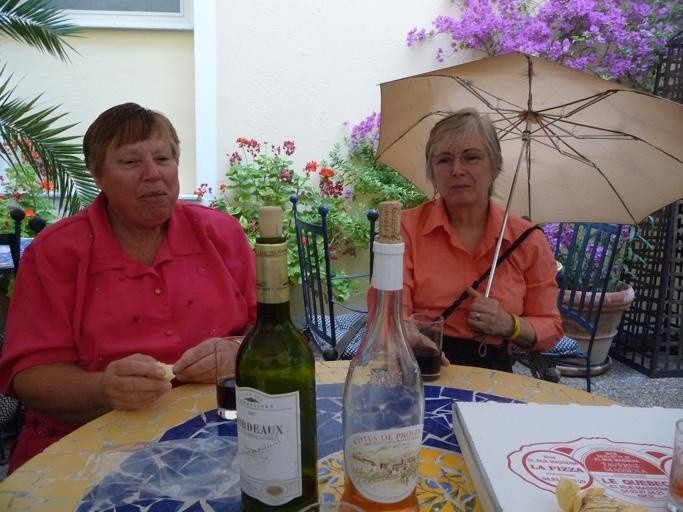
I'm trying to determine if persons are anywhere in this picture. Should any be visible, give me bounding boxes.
[0,100,258,477]
[361,106,565,375]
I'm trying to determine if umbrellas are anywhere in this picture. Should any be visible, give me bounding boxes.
[376,49,681,336]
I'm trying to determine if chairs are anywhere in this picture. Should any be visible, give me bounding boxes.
[0,208,26,465]
[288,193,623,394]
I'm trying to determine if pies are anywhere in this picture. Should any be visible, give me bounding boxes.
[557,478,648,512]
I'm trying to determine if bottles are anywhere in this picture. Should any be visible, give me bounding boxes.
[339,240,424,511]
[232,238,320,511]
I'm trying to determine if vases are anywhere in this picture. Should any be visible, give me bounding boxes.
[516,279,636,378]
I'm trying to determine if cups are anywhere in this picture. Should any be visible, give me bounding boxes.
[407,310,442,381]
[299,500,363,511]
[667,419,682,511]
[213,335,246,419]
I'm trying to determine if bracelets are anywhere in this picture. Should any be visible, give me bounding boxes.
[500,313,520,340]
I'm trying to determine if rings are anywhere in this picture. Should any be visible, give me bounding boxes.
[476,311,481,323]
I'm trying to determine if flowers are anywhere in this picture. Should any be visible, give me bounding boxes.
[406,1,683,293]
